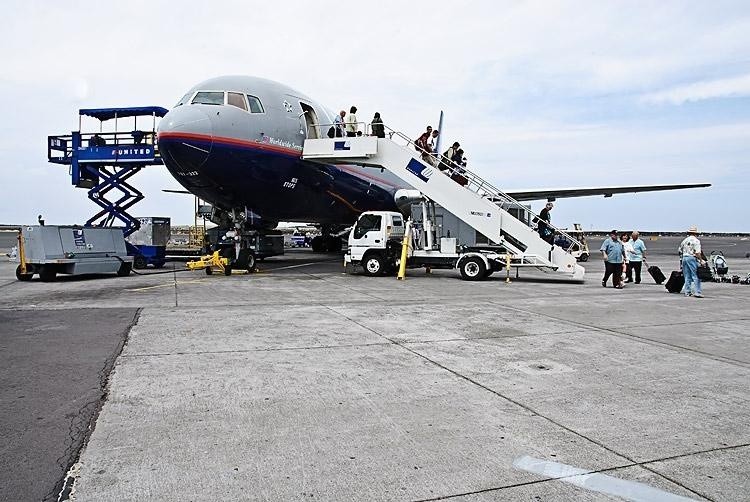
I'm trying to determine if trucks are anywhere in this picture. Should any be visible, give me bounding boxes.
[343,209,559,280]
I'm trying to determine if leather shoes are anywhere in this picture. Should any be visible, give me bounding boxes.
[623,277,633,283]
[602,281,626,289]
[635,280,640,284]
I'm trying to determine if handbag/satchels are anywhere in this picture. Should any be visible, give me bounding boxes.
[376,129,385,138]
[622,260,627,273]
[532,215,540,223]
[696,262,712,280]
[414,140,419,151]
[327,126,342,138]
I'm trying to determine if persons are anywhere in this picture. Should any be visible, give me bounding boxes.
[600,230,627,289]
[413,125,468,188]
[538,203,554,240]
[677,226,706,298]
[617,233,637,282]
[344,106,359,137]
[624,230,648,285]
[371,112,386,138]
[331,110,346,137]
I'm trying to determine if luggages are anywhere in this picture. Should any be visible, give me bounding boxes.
[643,260,666,285]
[664,271,685,294]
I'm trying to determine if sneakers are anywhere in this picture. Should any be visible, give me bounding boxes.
[684,291,693,297]
[694,292,705,298]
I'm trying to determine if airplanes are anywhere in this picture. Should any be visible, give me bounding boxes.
[157,75,711,281]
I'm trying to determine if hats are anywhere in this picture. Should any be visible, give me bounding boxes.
[686,225,701,233]
